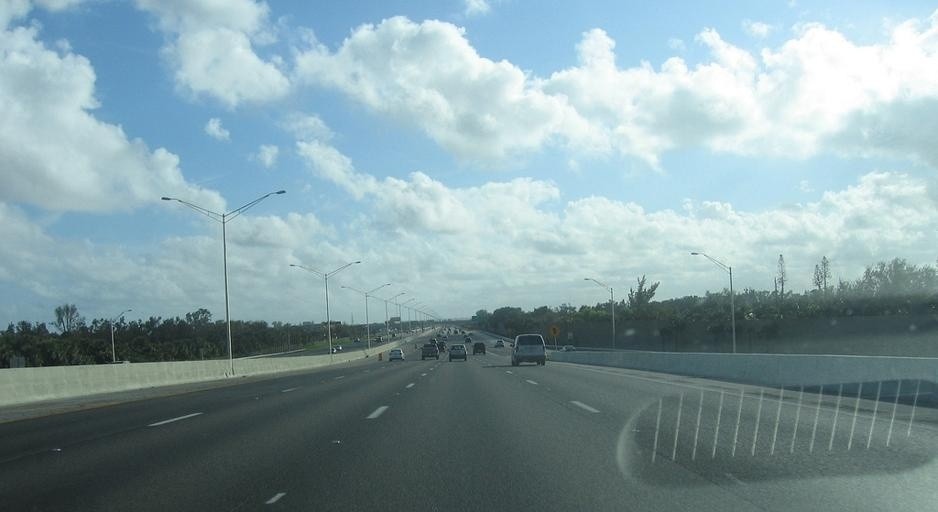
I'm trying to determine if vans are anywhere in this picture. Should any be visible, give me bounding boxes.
[509,333,548,368]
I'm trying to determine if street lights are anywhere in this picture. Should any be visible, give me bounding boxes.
[160,188,287,381]
[287,260,362,354]
[111,308,132,364]
[339,284,392,350]
[584,277,615,350]
[689,251,736,354]
[391,298,441,339]
[367,291,406,345]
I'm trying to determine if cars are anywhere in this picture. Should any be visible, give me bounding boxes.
[388,327,506,361]
[327,326,416,355]
[561,345,576,352]
